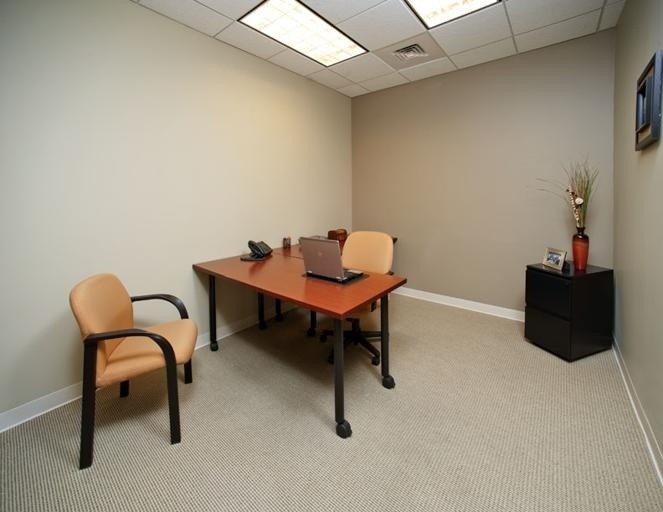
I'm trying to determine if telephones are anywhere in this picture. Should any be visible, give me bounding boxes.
[248,240,273,258]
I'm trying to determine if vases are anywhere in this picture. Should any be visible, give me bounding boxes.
[569,226,590,271]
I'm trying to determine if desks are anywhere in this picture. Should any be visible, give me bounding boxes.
[524,261,614,362]
[193,241,409,438]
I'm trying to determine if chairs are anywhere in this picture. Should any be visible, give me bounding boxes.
[68,271,198,469]
[322,230,395,365]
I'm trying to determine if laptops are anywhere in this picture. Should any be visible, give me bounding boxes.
[298,236,363,284]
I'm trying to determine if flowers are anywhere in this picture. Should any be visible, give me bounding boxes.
[535,161,600,229]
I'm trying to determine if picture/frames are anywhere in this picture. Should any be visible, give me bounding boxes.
[541,247,569,270]
[634,54,661,151]
